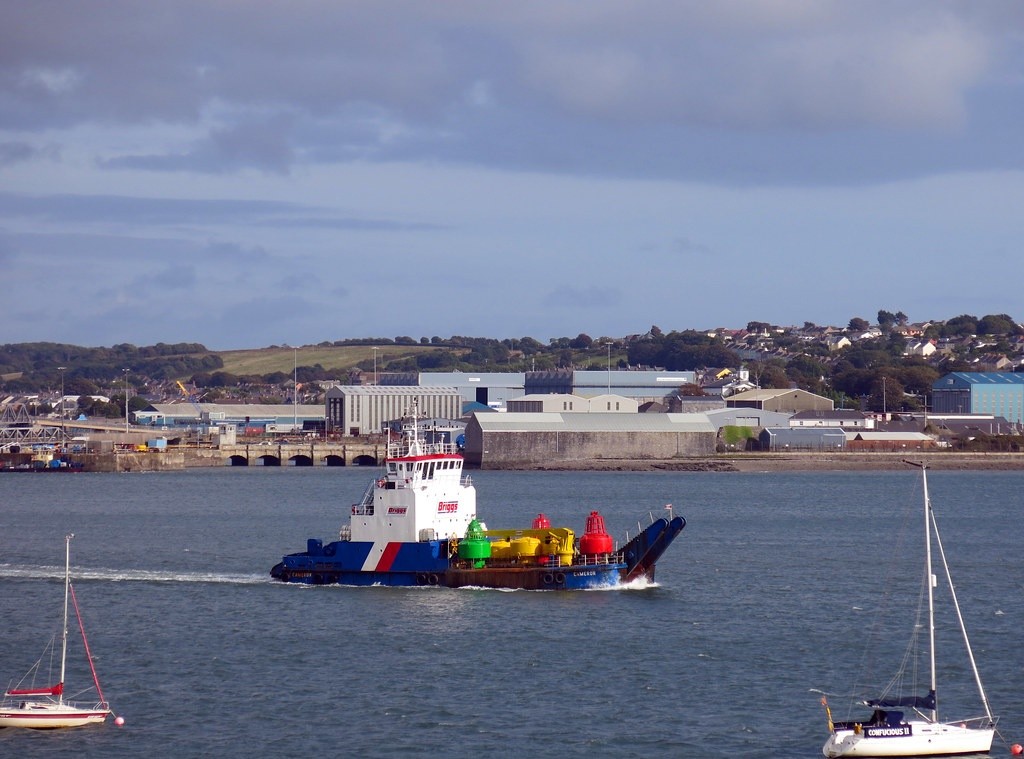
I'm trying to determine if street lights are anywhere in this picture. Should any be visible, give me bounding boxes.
[58,367,67,450]
[881,376,888,412]
[123,369,132,432]
[732,378,737,408]
[371,346,380,386]
[605,341,614,396]
[291,345,302,429]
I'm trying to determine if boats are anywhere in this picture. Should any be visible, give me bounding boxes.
[1,533,114,731]
[810,461,999,757]
[267,395,688,593]
